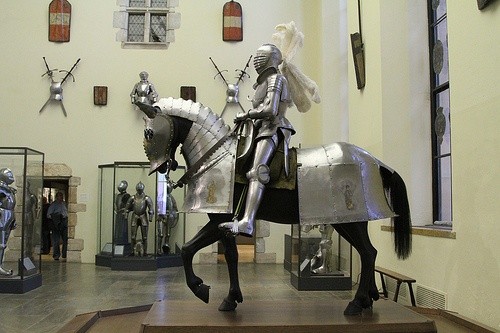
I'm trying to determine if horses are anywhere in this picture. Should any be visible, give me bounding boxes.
[132,96,413,317]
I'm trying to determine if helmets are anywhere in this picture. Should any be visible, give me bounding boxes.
[136,182,145,191]
[139,71,148,80]
[253,44,282,73]
[167,183,172,193]
[118,181,128,192]
[0,167,14,184]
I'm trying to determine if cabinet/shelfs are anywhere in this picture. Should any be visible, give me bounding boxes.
[0,146,45,293]
[289,221,353,290]
[94,161,188,270]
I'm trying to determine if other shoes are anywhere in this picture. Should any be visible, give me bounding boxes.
[62,252,67,257]
[53,256,59,261]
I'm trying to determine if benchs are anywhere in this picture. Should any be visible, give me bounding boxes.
[374,265,417,307]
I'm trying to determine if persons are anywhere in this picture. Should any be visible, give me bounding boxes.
[311,224,335,276]
[42,192,68,260]
[0,168,17,276]
[24,180,39,260]
[158,182,179,254]
[217,43,296,239]
[124,182,154,255]
[114,180,134,245]
